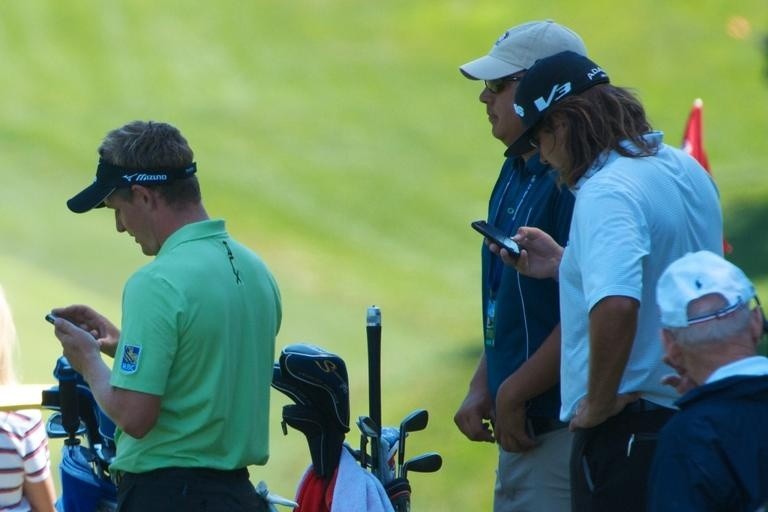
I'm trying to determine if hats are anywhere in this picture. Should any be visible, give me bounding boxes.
[504,50,610,158]
[655,250,757,330]
[458,19,588,84]
[66,158,200,215]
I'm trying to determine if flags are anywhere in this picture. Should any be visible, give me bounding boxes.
[682,107,732,254]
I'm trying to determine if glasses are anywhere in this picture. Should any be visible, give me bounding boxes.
[482,76,527,94]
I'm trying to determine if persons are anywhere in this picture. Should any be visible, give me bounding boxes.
[53,118,284,512]
[0,286,58,512]
[643,248,767,512]
[501,49,727,511]
[454,19,589,511]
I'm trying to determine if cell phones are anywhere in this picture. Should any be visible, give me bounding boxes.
[44,314,98,341]
[471,218,524,259]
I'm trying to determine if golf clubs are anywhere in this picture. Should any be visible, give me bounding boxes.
[382,430,400,480]
[274,366,319,475]
[402,454,443,480]
[396,410,427,478]
[283,346,350,474]
[34,362,113,488]
[358,417,377,469]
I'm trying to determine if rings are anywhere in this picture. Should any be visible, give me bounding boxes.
[574,408,579,416]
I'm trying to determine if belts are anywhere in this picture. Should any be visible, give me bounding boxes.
[492,413,570,437]
[619,397,661,414]
